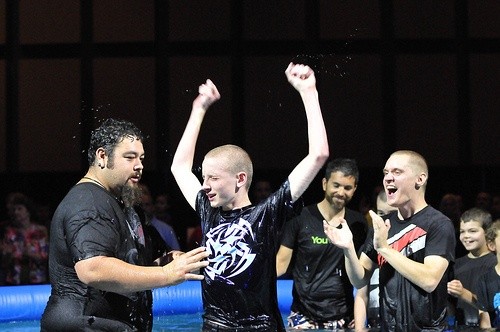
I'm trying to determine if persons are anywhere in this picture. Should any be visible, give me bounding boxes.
[171,61,329,332]
[41,118,209,332]
[0,151,500,332]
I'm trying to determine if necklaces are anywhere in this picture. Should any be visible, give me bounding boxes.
[83,176,124,204]
